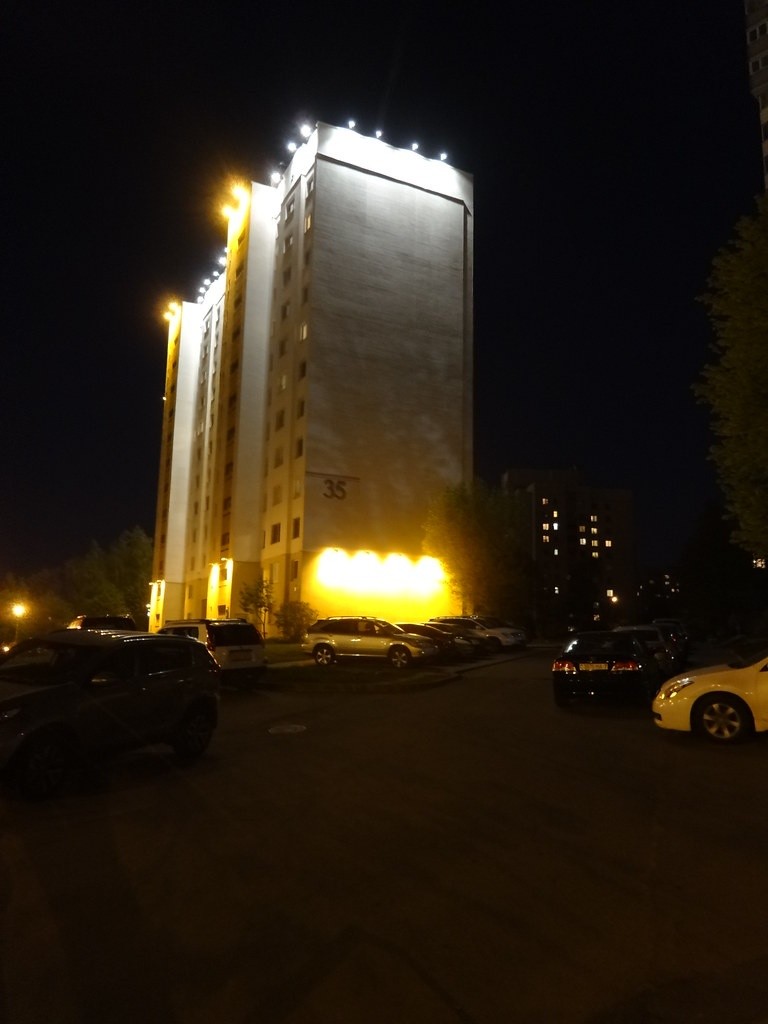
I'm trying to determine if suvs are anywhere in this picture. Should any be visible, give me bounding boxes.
[156,618,269,687]
[0,631,220,795]
[301,615,440,668]
[68,614,136,632]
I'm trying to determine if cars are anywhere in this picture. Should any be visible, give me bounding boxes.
[551,620,767,745]
[397,614,529,657]
